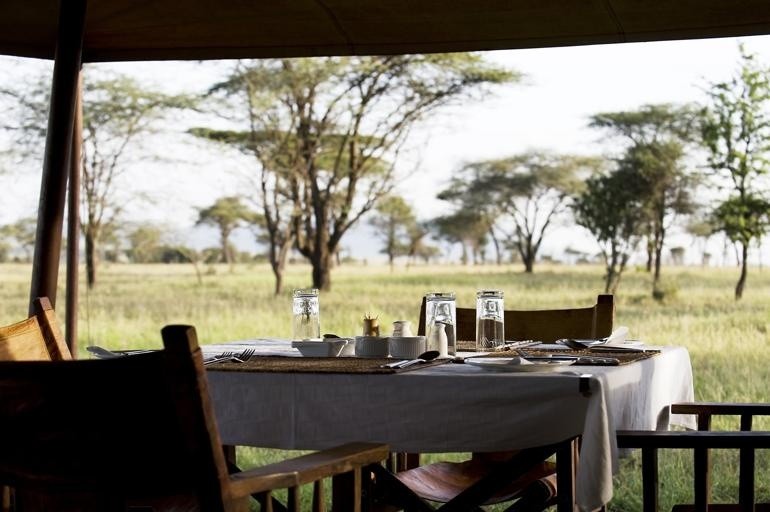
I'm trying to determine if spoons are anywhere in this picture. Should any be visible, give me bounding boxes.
[396,350,441,370]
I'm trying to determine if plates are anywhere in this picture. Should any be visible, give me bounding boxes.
[462,355,575,371]
[556,338,644,348]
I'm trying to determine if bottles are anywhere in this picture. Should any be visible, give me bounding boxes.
[360,311,381,337]
[392,319,412,337]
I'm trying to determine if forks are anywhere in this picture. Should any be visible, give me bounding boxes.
[202,346,256,368]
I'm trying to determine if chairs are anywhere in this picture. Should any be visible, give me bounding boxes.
[393,295,615,510]
[615,403,769,511]
[0,296,73,362]
[0,325,389,512]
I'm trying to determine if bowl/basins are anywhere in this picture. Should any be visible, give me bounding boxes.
[355,333,388,358]
[389,334,426,360]
[290,335,355,357]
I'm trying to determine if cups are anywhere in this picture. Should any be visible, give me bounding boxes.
[422,291,459,357]
[476,287,506,348]
[293,288,321,340]
